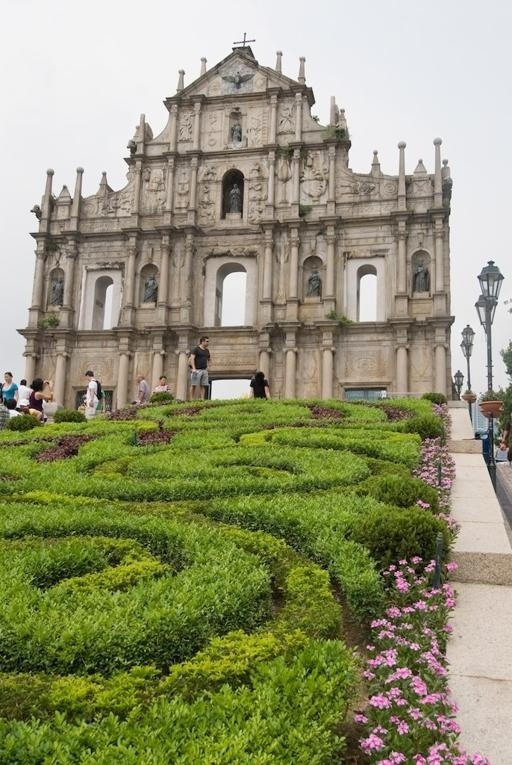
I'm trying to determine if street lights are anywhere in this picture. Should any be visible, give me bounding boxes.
[453,256,506,494]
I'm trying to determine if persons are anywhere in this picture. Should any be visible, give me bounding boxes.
[0,372,53,429]
[231,121,241,142]
[144,272,158,302]
[250,372,271,399]
[136,375,169,406]
[415,255,429,291]
[85,371,99,419]
[50,278,63,304]
[229,184,241,213]
[307,263,320,296]
[190,336,210,400]
[494,412,512,462]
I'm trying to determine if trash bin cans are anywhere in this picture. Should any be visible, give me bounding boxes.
[475,432,490,454]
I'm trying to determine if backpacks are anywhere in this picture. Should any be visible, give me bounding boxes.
[92,380,103,400]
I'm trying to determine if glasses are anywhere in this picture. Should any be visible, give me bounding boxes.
[204,340,209,342]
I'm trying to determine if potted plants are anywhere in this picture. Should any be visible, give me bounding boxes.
[478,394,503,412]
[462,389,475,401]
[47,315,58,328]
[468,398,476,403]
[481,403,505,418]
